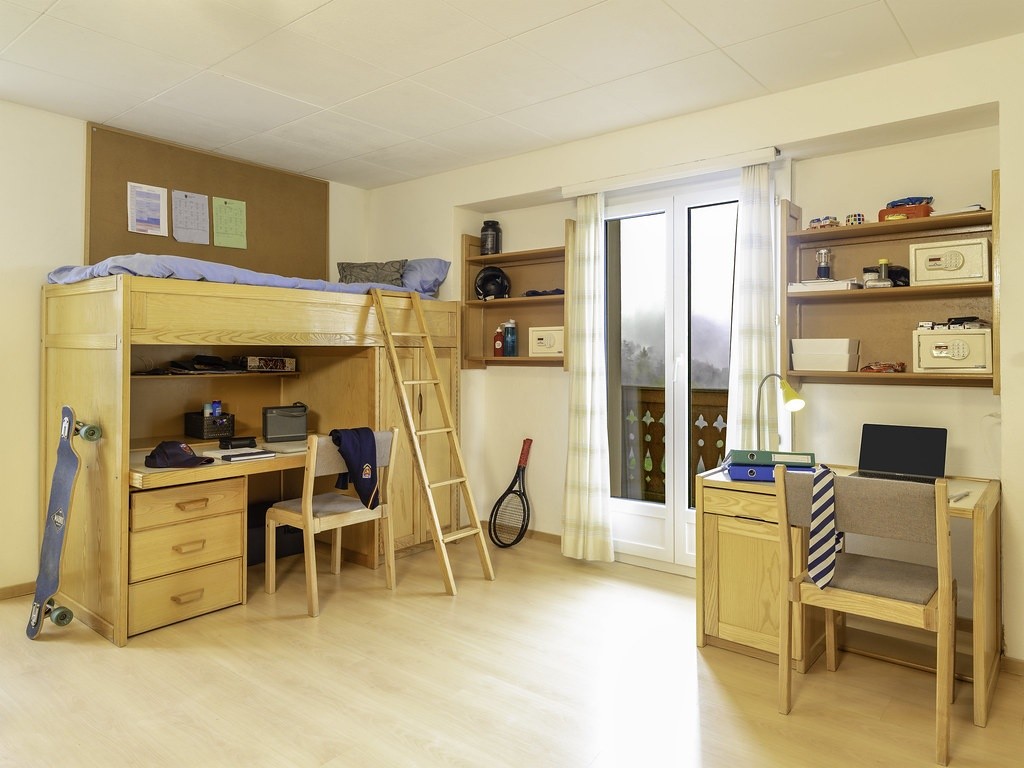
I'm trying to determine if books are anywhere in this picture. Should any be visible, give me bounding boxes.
[203,446,276,462]
[930,206,986,217]
[788,282,861,292]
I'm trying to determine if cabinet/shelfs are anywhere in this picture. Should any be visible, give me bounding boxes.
[128,475,248,640]
[776,168,1000,395]
[127,344,304,453]
[460,219,578,369]
[282,347,461,570]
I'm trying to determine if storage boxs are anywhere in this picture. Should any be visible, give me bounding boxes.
[184,411,235,439]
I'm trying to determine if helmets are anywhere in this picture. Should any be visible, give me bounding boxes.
[475,266,510,299]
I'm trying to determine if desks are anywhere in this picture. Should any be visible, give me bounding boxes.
[695,463,1002,728]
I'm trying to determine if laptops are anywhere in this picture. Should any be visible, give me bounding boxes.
[849,423,947,484]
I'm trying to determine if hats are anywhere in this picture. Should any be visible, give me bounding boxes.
[145,441,214,468]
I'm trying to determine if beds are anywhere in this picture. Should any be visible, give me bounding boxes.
[40,249,456,344]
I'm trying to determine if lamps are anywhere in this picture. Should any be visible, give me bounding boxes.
[756,372,805,452]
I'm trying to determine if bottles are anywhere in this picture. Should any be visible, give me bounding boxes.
[204,404,213,418]
[816,249,830,279]
[500,319,517,357]
[494,327,504,357]
[879,259,888,281]
[212,401,222,417]
[480,221,502,256]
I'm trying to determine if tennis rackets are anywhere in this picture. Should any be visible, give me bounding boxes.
[488,437,534,547]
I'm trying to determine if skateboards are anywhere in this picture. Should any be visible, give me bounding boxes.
[26,406,101,641]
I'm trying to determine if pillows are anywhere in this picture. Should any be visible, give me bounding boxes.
[397,258,452,295]
[334,259,407,287]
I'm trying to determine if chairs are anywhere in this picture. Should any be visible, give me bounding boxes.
[259,425,401,619]
[772,465,956,766]
